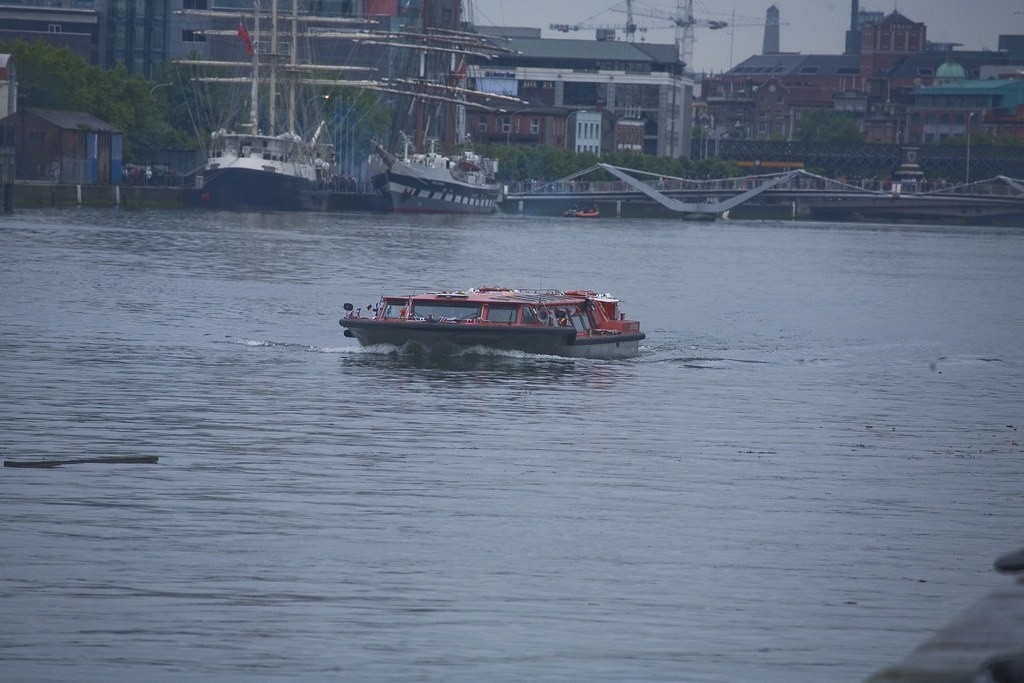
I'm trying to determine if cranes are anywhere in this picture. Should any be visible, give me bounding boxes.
[675,0,695,74]
[611,6,790,30]
[549,0,728,43]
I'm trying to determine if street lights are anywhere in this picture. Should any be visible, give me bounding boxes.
[565,110,586,149]
[507,109,532,145]
[967,112,974,183]
[614,115,641,152]
[700,125,709,159]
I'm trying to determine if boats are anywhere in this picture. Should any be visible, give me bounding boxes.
[339,285,646,359]
[564,208,599,217]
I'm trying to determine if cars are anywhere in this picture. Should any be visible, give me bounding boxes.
[121,166,177,186]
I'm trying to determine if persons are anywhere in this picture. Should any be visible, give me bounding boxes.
[524,177,581,192]
[658,176,670,190]
[874,176,878,190]
[901,177,946,194]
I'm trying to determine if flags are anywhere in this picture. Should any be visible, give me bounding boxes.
[238,22,252,54]
[400,306,407,317]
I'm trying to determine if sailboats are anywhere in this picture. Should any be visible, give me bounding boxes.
[171,0,379,211]
[351,0,528,214]
